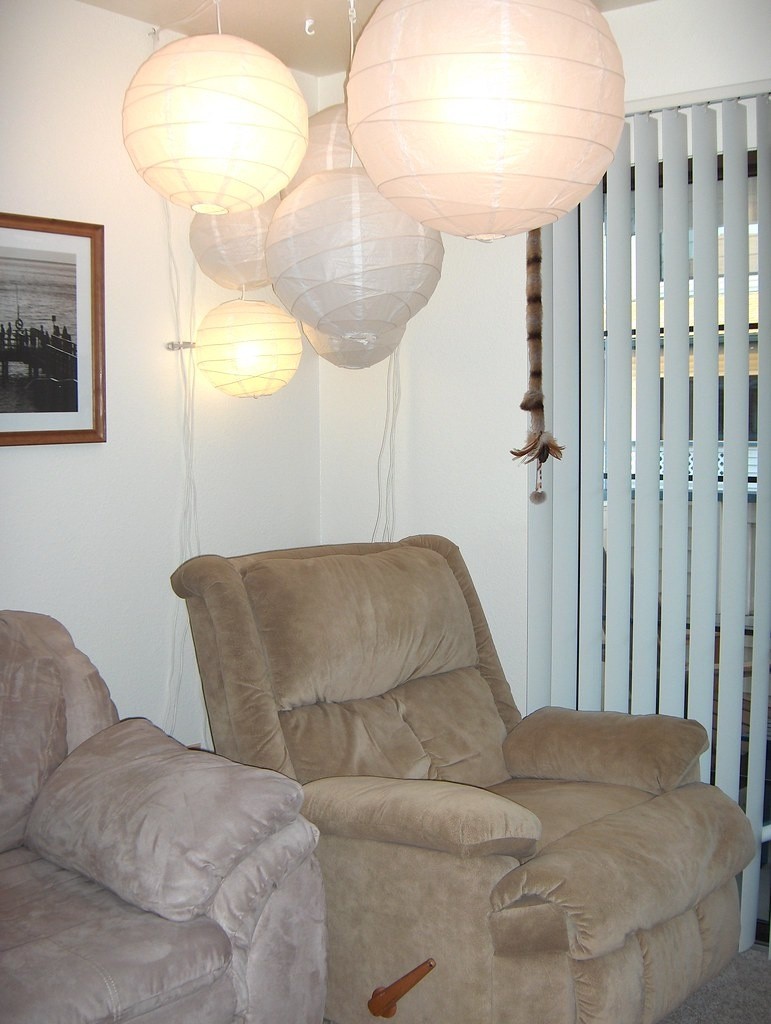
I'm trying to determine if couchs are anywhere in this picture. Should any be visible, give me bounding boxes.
[0,610,329,1024]
[171,534,759,1024]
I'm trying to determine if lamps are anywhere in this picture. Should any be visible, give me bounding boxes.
[123,0,625,400]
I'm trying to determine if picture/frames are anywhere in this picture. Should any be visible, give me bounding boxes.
[0,211,107,446]
